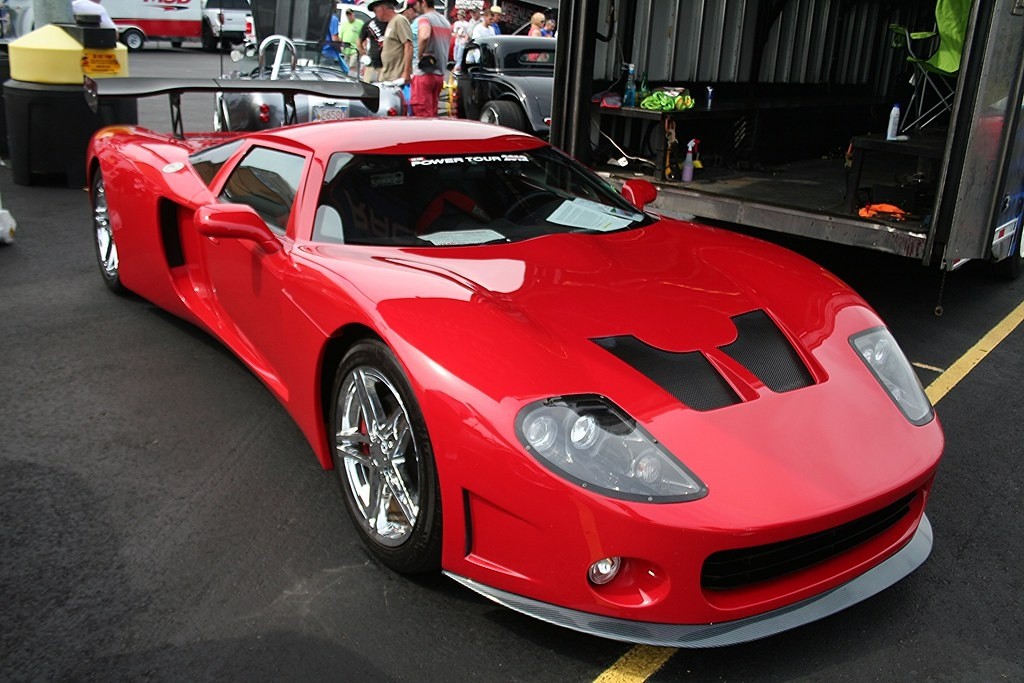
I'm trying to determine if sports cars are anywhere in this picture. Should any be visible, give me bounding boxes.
[212,36,402,141]
[86,117,943,650]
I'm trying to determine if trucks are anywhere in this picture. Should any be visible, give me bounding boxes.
[99,0,256,53]
[553,1,1024,284]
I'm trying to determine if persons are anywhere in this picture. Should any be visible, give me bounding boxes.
[401,0,452,117]
[541,19,556,37]
[368,0,414,118]
[321,4,349,74]
[451,6,506,74]
[72,0,119,40]
[528,12,547,62]
[338,8,367,76]
[357,17,388,83]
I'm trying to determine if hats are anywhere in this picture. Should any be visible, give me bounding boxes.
[468,4,478,11]
[368,0,399,11]
[400,0,416,13]
[346,8,353,14]
[491,6,505,15]
[456,10,466,18]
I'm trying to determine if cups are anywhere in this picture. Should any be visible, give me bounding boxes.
[705,87,714,109]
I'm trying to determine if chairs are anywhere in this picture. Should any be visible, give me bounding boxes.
[888,0,975,131]
[424,178,487,236]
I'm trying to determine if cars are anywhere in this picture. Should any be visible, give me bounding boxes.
[445,34,558,140]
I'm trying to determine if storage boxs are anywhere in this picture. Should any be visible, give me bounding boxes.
[55,23,116,50]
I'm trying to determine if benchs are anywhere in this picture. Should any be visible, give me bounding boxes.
[587,74,958,185]
[844,122,952,207]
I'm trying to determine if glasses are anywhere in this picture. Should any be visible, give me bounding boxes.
[541,20,546,24]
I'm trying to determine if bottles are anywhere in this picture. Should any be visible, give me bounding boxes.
[681,139,700,182]
[887,104,899,140]
[639,72,649,102]
[622,63,636,108]
